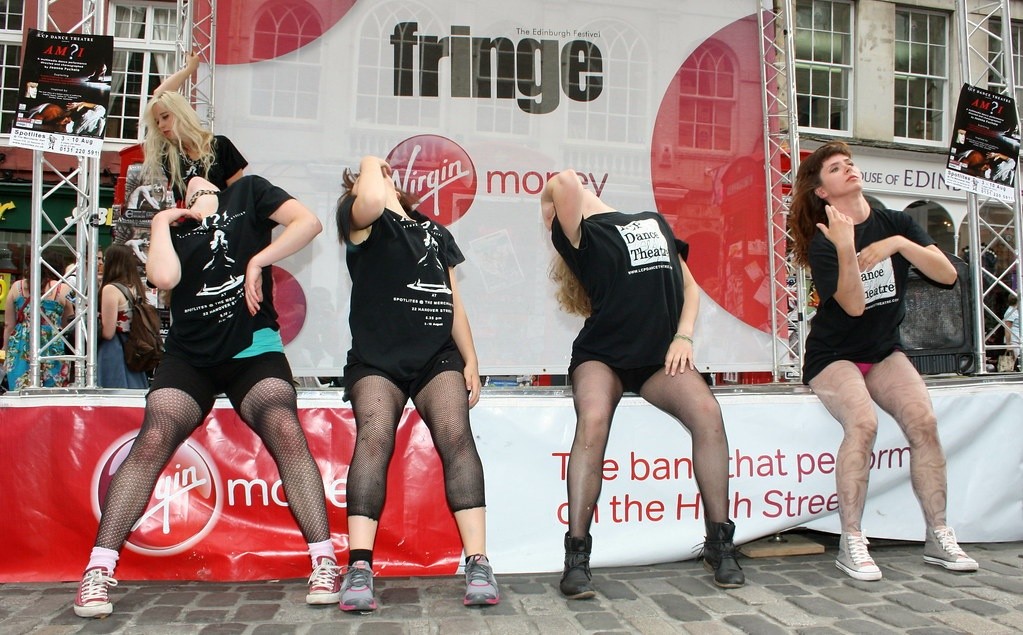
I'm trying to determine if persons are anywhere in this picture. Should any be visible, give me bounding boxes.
[719,134,764,196]
[334,155,501,613]
[137,51,249,225]
[786,140,983,583]
[26,100,106,137]
[954,149,1016,189]
[2,251,74,392]
[125,179,164,210]
[127,232,151,266]
[81,35,113,84]
[96,242,151,388]
[65,242,103,304]
[539,168,746,600]
[71,174,347,619]
[999,100,1019,136]
[1000,291,1022,371]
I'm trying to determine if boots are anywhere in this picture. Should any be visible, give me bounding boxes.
[558,531,596,599]
[702,514,746,589]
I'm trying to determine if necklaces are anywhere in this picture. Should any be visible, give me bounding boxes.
[184,190,219,210]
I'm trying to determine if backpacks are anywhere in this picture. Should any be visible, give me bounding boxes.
[111,280,166,373]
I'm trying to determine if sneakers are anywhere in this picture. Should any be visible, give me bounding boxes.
[923,526,980,572]
[306,556,344,604]
[835,531,884,581]
[338,567,376,611]
[74,566,113,617]
[464,559,498,606]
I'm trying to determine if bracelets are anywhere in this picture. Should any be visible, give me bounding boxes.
[672,334,693,345]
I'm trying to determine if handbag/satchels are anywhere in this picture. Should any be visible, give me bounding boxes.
[996,350,1016,372]
